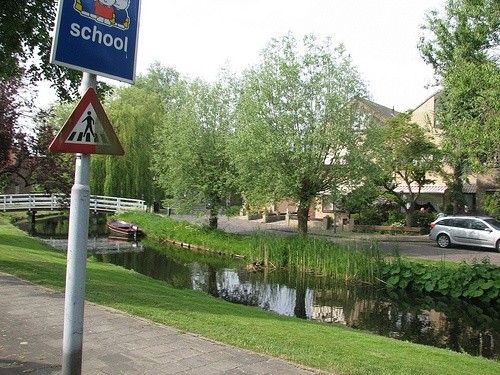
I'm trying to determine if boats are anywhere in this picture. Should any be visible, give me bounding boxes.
[105,217,142,241]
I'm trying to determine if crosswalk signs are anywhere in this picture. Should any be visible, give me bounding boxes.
[47,87,127,155]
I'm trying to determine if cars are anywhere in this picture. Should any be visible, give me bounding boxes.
[429,215,500,252]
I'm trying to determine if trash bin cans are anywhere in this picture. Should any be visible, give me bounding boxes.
[322,216,331,230]
[209,217,218,229]
